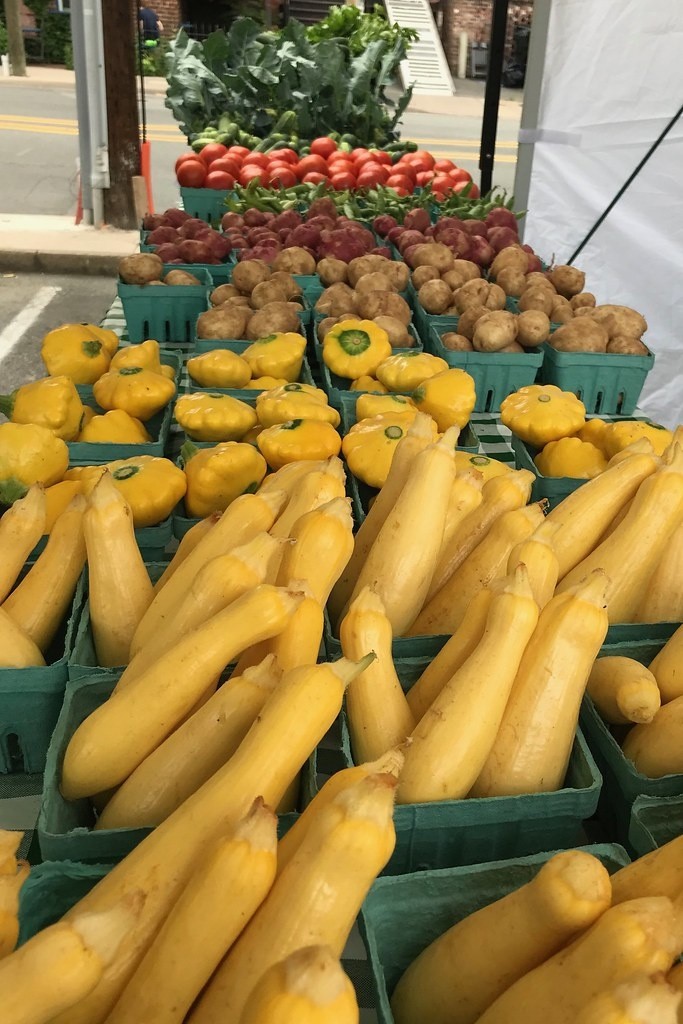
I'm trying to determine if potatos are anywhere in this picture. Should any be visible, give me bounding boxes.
[118,249,651,357]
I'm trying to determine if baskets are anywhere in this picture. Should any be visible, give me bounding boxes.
[1,189,683,1023]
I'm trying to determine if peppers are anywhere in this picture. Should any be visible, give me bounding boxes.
[224,175,526,224]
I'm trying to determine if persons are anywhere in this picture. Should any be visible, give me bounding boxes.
[138,6,164,39]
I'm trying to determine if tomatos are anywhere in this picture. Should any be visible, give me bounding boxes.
[176,139,480,201]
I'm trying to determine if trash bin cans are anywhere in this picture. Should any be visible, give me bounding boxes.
[470,41,489,77]
[511,24,530,77]
